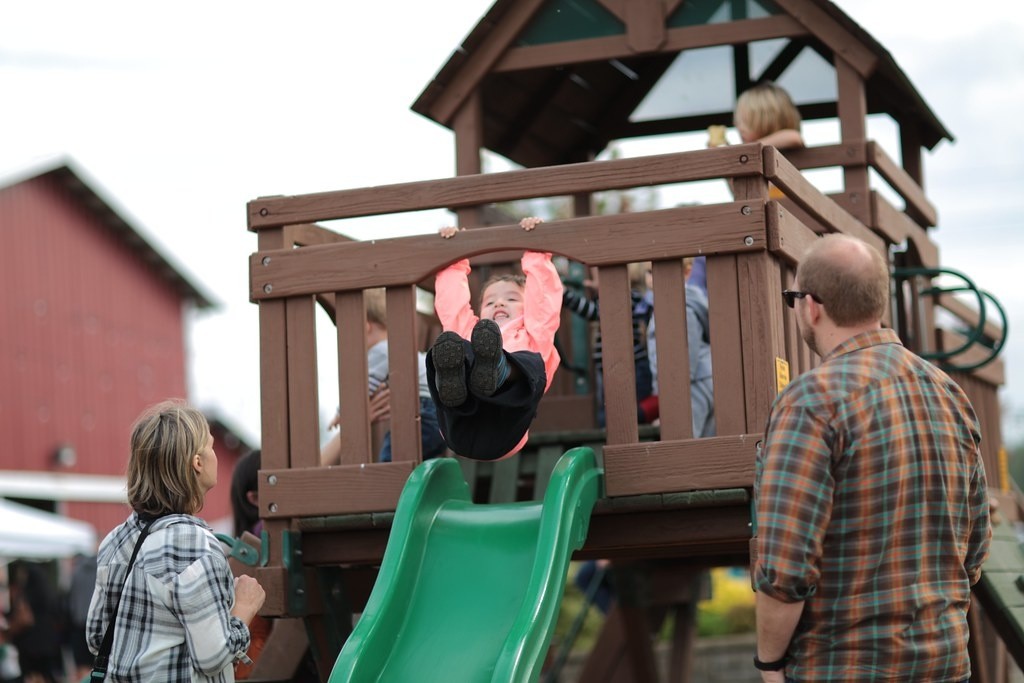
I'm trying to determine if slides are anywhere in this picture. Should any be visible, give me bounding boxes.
[326,446,602,682]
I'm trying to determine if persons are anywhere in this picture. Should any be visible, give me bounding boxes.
[13,553,96,683]
[647,256,711,437]
[750,235,992,683]
[329,288,444,463]
[574,558,610,613]
[707,80,805,201]
[562,264,650,426]
[425,217,563,461]
[86,400,265,683]
[228,384,390,539]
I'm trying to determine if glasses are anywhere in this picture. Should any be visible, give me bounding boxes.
[782,286,824,308]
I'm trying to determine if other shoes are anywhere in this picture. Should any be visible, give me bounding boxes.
[433,319,506,404]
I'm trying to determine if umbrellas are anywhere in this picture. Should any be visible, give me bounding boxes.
[0,502,92,560]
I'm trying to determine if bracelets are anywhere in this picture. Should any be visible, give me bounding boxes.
[753,657,788,671]
